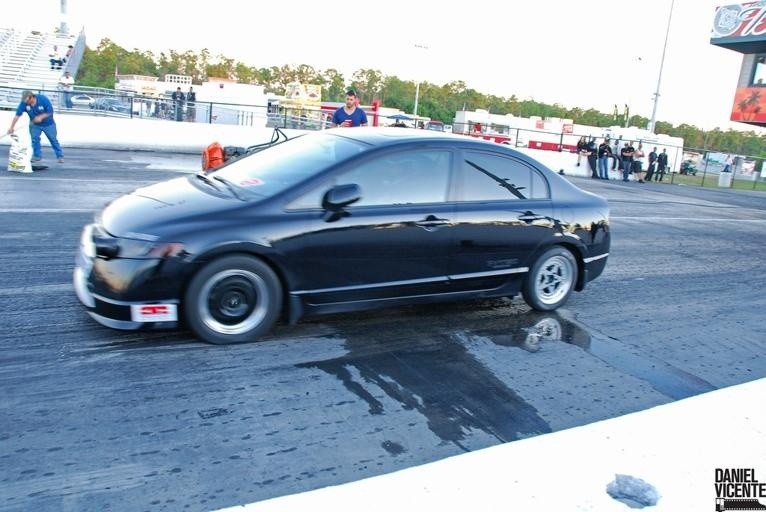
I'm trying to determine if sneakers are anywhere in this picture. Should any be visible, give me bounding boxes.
[31,159,39,162]
[59,159,64,163]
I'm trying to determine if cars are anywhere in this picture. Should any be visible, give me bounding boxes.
[72,93,130,114]
[72,124,611,347]
[680,158,699,176]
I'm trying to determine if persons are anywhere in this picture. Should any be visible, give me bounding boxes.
[353,94,360,107]
[48,44,63,69]
[6,91,65,162]
[723,155,733,172]
[57,72,75,108]
[134,86,196,122]
[643,147,656,181]
[576,134,645,184]
[63,46,73,62]
[472,122,481,133]
[654,148,667,181]
[266,108,333,131]
[391,118,405,127]
[330,92,368,129]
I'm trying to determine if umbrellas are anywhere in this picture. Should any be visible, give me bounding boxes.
[387,114,411,120]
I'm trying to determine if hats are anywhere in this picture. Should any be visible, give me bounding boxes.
[21,91,31,102]
